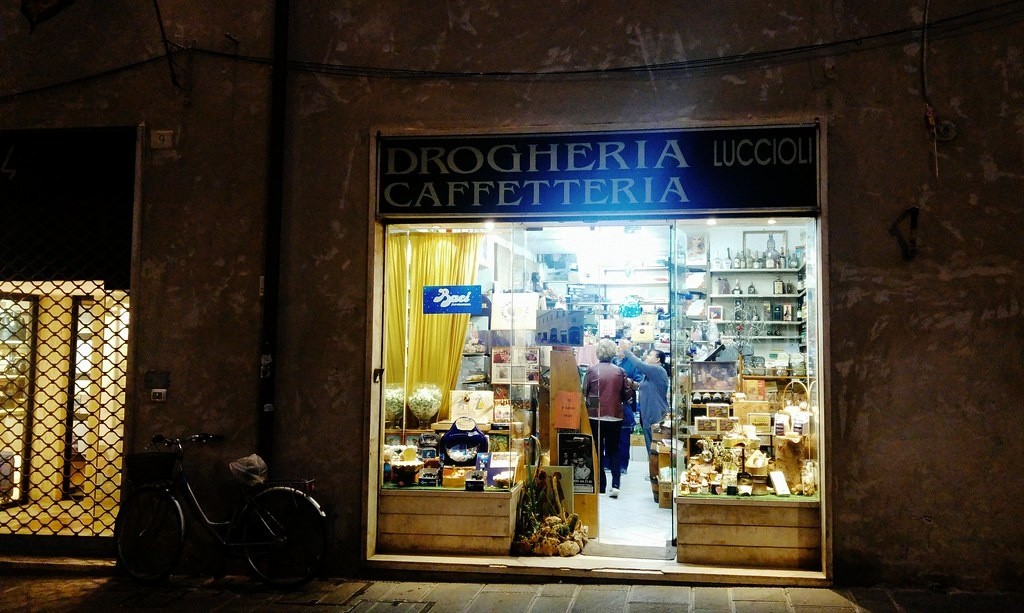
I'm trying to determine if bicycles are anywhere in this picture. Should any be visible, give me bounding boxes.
[112,432,331,590]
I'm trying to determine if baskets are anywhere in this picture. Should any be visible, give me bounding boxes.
[125,451,176,489]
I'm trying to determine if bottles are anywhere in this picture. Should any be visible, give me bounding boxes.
[797,274,805,294]
[693,391,732,404]
[712,233,798,268]
[748,281,755,294]
[773,274,784,294]
[732,279,742,294]
[796,303,802,321]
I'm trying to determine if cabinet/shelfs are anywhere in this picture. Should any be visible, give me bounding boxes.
[377,256,824,571]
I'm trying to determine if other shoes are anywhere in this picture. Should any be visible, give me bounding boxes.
[620,469,627,475]
[645,476,650,481]
[609,488,620,498]
[605,467,611,473]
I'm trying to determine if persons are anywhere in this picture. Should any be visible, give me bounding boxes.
[604,339,643,476]
[619,339,670,481]
[561,451,591,480]
[643,349,652,360]
[583,339,632,498]
[689,236,705,255]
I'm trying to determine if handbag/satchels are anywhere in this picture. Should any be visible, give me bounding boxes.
[437,417,488,466]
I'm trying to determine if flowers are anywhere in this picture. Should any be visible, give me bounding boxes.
[408,382,443,420]
[385,382,404,423]
